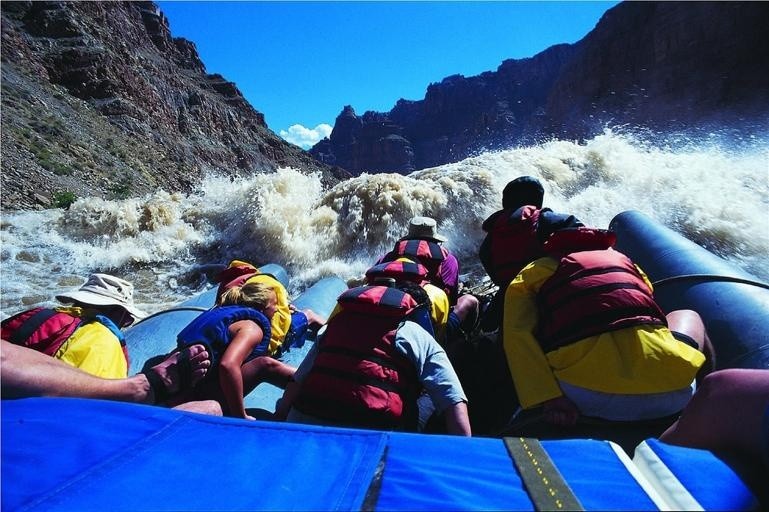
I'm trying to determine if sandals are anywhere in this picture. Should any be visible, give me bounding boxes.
[136,341,214,405]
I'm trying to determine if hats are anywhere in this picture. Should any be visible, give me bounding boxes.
[401,216,449,242]
[258,263,289,289]
[55,274,149,319]
[503,176,544,209]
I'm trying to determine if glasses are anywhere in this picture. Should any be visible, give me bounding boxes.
[122,315,134,327]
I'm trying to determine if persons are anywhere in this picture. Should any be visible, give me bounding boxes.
[0,339,214,406]
[176,281,298,422]
[365,256,479,346]
[273,281,471,438]
[0,274,145,379]
[215,260,326,358]
[503,227,716,434]
[374,216,459,305]
[478,177,585,284]
